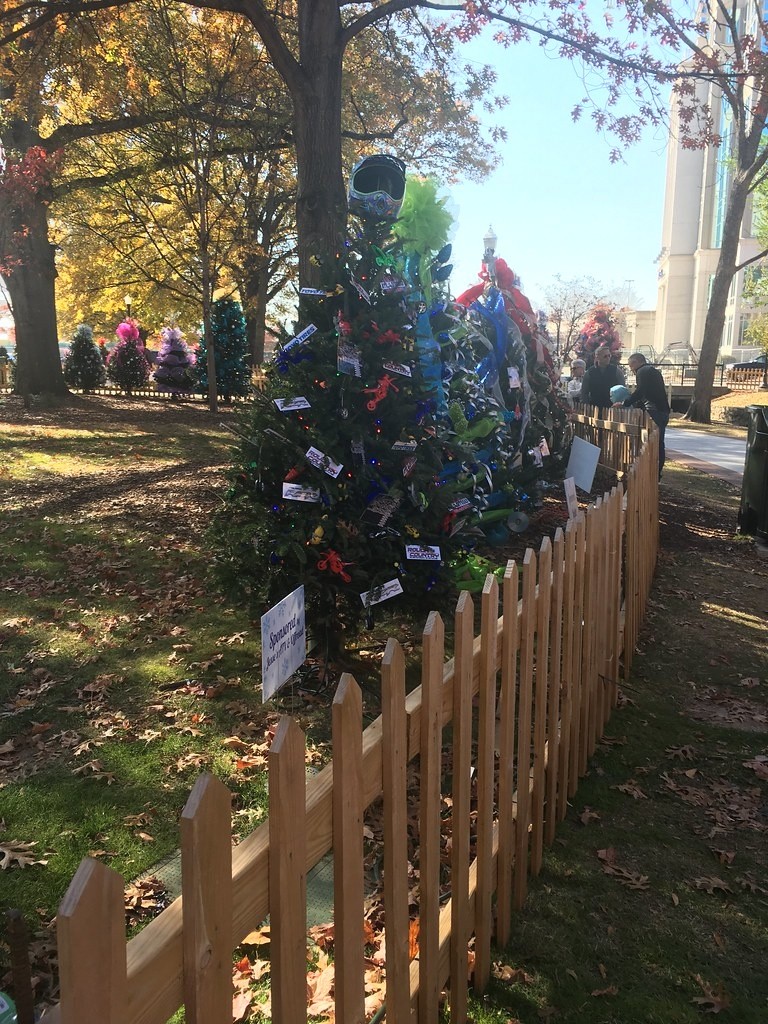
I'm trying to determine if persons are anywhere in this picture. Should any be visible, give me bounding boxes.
[582,347,625,448]
[568,359,586,403]
[613,353,670,484]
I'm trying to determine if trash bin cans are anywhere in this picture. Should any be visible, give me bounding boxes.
[737,404,768,543]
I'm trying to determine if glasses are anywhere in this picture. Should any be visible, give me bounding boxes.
[598,354,611,358]
[571,367,582,370]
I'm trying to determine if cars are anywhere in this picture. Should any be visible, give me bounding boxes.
[726,354,768,382]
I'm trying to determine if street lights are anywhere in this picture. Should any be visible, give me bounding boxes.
[125,295,134,321]
[625,279,635,308]
